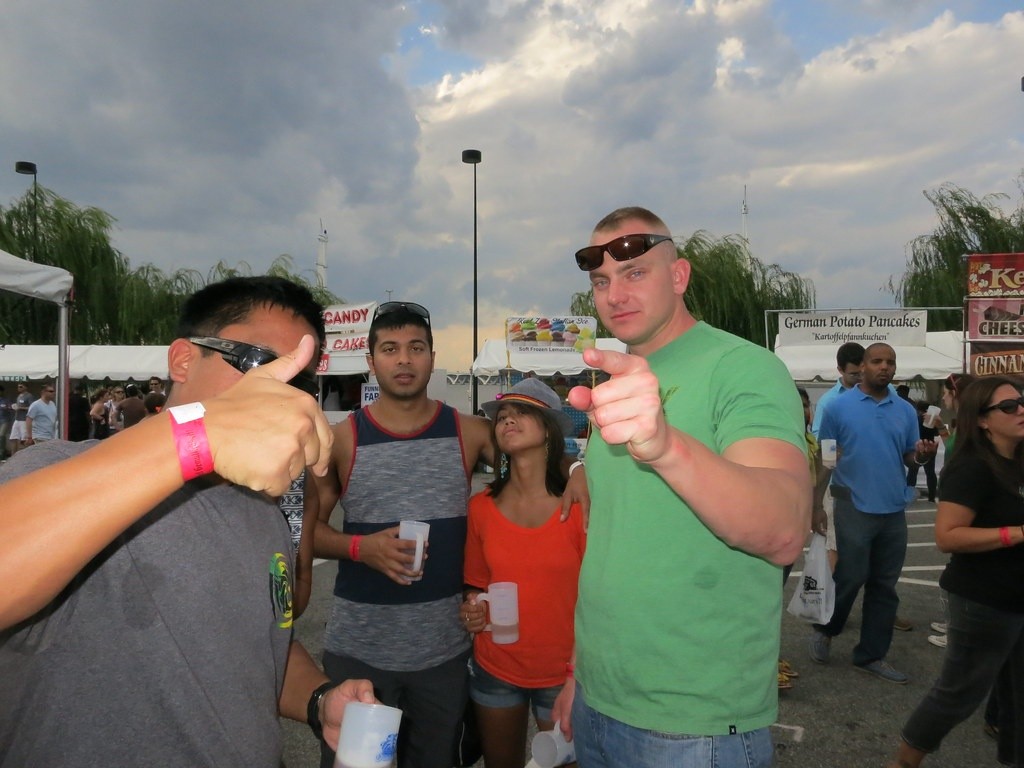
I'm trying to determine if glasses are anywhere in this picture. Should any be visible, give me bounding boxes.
[150,382,159,386]
[18,386,24,389]
[842,372,861,378]
[115,391,124,393]
[373,302,431,326]
[983,397,1024,413]
[575,233,674,271]
[187,336,320,400]
[47,390,54,393]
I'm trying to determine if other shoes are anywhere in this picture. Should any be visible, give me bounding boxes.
[927,499,935,503]
[985,721,1000,739]
[893,617,913,630]
[996,758,1024,768]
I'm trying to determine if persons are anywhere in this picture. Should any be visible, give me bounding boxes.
[0,276,385,768]
[893,377,1024,768]
[313,301,592,768]
[280,469,319,622]
[778,342,974,689]
[460,378,587,768]
[552,206,812,768]
[0,376,173,459]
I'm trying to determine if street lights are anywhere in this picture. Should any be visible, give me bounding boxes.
[15,160,40,345]
[461,149,482,414]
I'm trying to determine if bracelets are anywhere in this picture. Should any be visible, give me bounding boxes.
[569,461,585,476]
[913,456,929,465]
[999,526,1024,547]
[349,535,361,560]
[814,505,823,512]
[307,680,339,740]
[939,426,948,436]
[167,402,215,481]
[566,663,575,679]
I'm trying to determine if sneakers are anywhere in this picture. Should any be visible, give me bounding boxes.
[811,631,830,663]
[854,660,908,684]
[928,634,947,647]
[931,622,947,633]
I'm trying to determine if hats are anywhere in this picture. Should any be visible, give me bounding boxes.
[480,377,575,438]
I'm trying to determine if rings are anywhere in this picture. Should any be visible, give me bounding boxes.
[465,613,469,621]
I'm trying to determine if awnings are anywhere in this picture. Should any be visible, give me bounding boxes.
[472,338,626,377]
[0,344,171,381]
[774,331,970,382]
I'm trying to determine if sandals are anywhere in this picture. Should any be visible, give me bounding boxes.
[778,673,793,688]
[779,660,797,676]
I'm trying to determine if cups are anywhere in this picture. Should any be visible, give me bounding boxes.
[332,701,403,768]
[820,439,837,470]
[399,520,431,581]
[922,405,941,429]
[474,581,520,644]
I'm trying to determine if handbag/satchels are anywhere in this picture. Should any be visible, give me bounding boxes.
[786,530,835,625]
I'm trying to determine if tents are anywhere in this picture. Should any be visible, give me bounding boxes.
[0,250,74,437]
